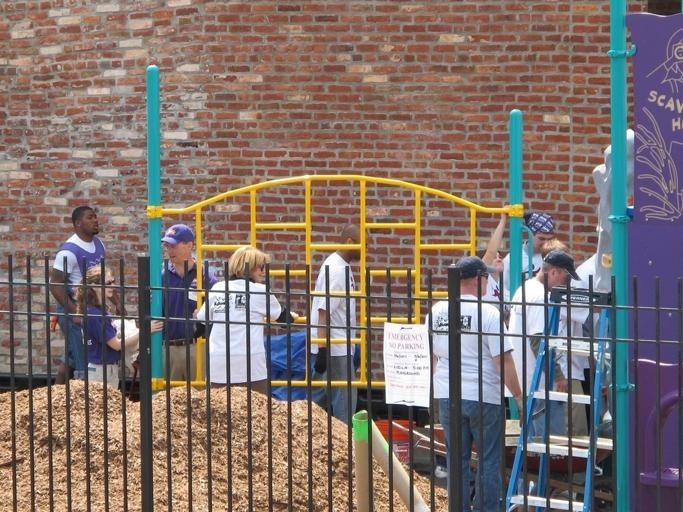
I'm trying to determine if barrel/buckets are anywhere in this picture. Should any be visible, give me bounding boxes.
[376,419,416,465]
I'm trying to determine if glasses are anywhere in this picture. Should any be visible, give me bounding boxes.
[91,278,117,286]
[478,273,489,281]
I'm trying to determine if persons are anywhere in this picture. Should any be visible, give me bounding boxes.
[473,206,511,332]
[158,223,221,382]
[46,206,107,388]
[497,207,556,295]
[553,250,614,479]
[504,248,582,442]
[424,253,528,512]
[75,264,163,391]
[305,222,373,426]
[192,242,300,400]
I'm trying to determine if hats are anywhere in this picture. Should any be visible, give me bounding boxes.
[161,222,194,246]
[542,250,581,280]
[456,256,498,279]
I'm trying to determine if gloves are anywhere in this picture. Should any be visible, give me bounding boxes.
[313,347,329,373]
[415,407,432,427]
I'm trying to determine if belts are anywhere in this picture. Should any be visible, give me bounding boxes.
[163,338,202,348]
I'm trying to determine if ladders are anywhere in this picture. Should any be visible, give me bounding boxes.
[506,285,614,512]
